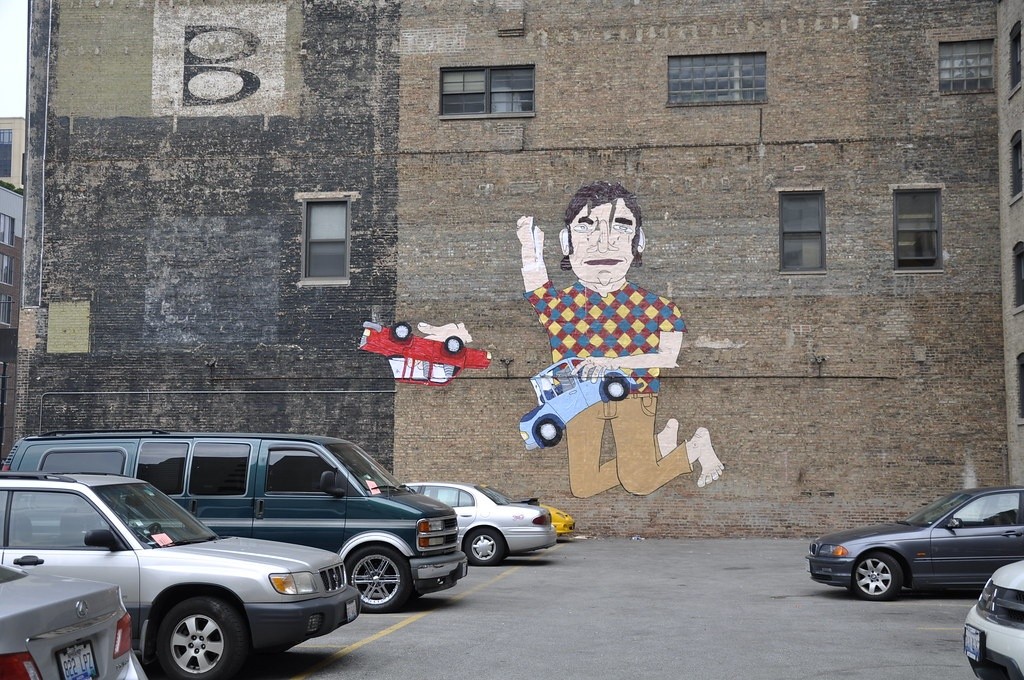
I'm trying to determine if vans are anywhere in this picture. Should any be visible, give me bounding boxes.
[0,428,468,615]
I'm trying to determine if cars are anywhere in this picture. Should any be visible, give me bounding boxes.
[804,487,1024,602]
[0,563,150,679]
[479,484,576,538]
[962,558,1024,680]
[403,482,557,568]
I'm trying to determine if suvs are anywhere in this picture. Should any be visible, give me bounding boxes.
[0,468,362,679]
